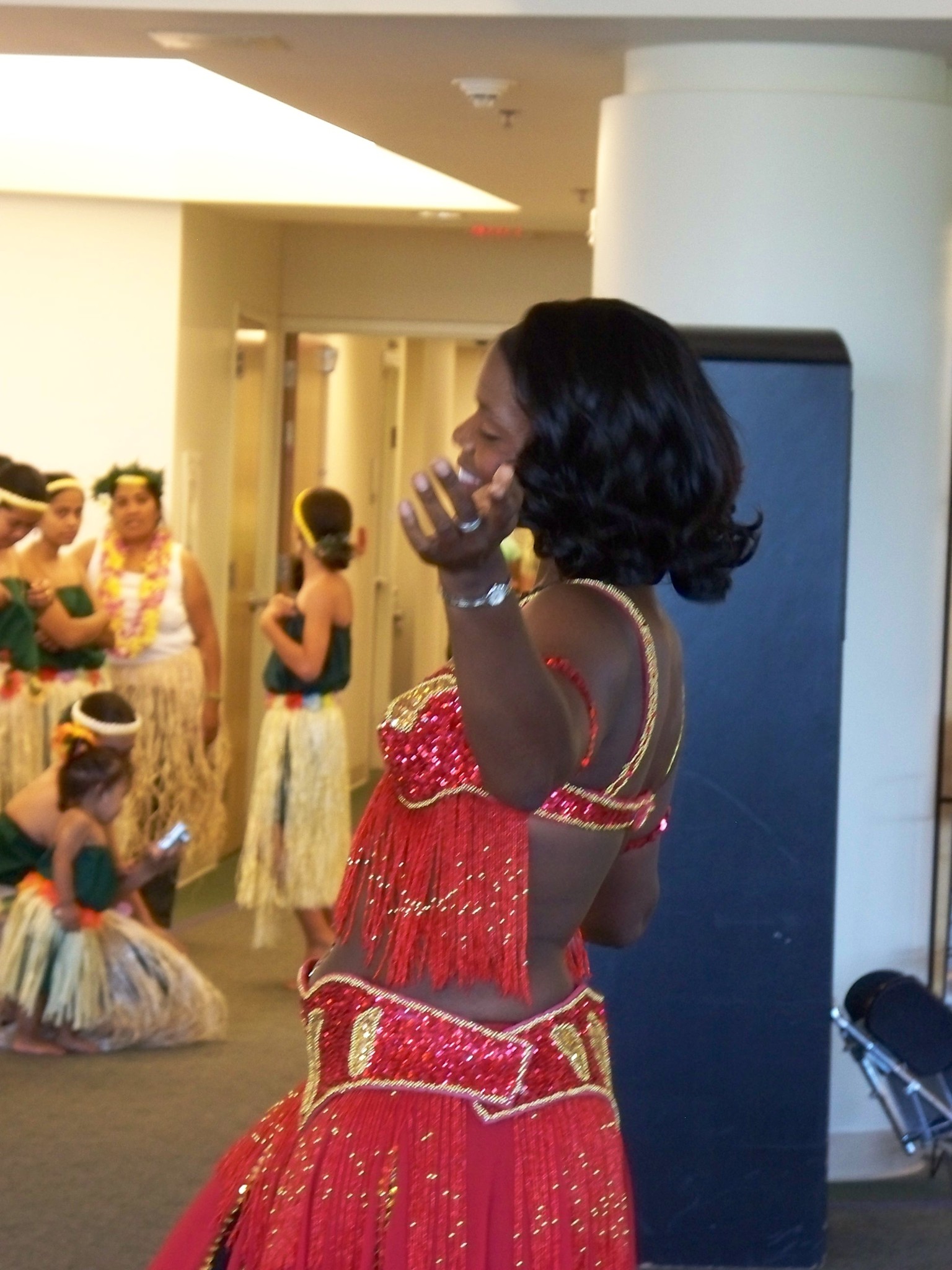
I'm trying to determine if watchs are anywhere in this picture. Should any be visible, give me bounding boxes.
[441,573,512,607]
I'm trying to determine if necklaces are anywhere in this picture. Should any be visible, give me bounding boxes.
[100,523,169,660]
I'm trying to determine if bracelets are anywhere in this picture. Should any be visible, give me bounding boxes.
[204,690,221,702]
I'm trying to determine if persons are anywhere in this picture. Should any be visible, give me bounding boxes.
[143,297,764,1270]
[0,452,226,1059]
[72,460,222,931]
[232,487,353,988]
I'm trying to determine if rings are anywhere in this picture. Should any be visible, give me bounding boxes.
[46,589,52,595]
[42,580,50,586]
[456,515,482,533]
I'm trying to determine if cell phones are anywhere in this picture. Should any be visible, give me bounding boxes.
[156,821,190,849]
